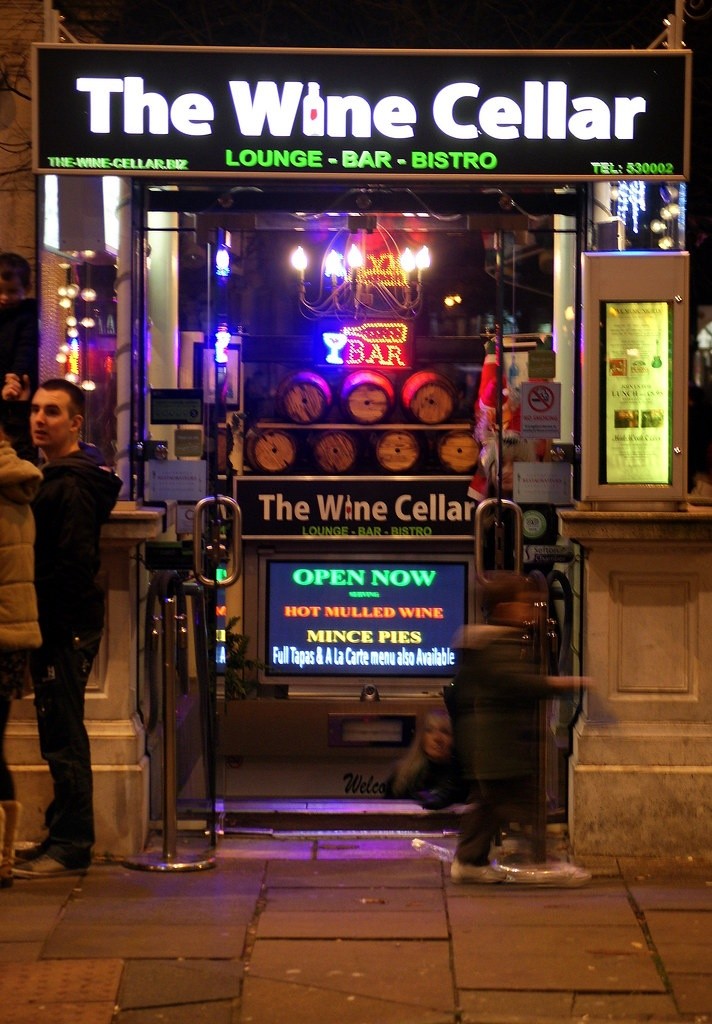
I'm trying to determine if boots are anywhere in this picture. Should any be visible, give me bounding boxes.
[0,801,22,889]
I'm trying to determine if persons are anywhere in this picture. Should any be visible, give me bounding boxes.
[0,252,124,886]
[389,713,463,811]
[450,573,597,887]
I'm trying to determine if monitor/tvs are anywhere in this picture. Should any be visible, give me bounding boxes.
[257,553,477,702]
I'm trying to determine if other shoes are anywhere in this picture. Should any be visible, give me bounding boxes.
[14,844,45,861]
[451,857,507,883]
[10,854,91,877]
[502,860,592,888]
